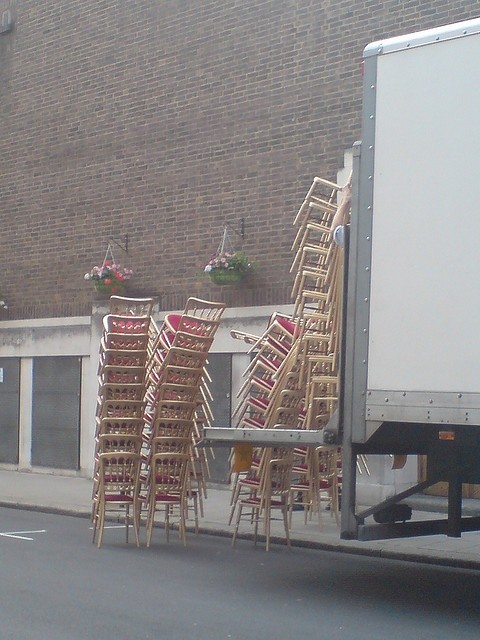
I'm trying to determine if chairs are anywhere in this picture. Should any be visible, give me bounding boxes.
[139,294,228,549]
[90,293,158,550]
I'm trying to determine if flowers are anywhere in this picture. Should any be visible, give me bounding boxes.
[82,262,133,293]
[201,253,248,273]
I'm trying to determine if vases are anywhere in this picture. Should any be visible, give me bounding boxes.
[207,267,241,285]
[91,278,125,294]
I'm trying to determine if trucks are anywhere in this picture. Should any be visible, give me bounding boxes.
[195,14,480,541]
